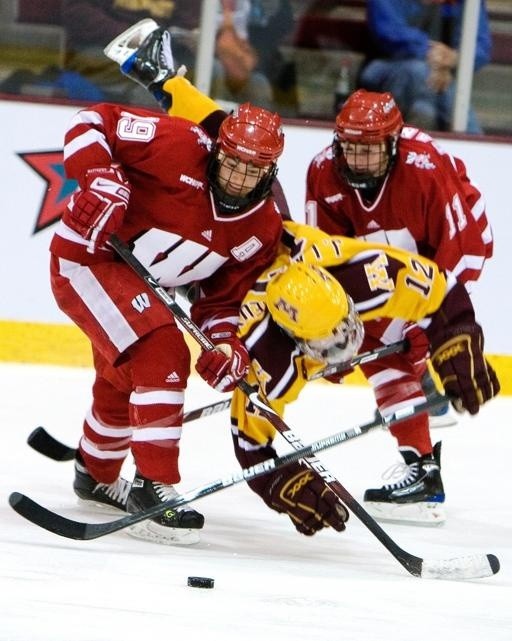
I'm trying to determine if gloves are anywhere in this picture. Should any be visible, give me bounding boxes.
[427,320,499,416]
[197,334,250,393]
[264,460,352,536]
[64,168,132,255]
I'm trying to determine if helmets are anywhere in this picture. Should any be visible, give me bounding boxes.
[211,102,285,214]
[266,260,364,367]
[333,87,405,190]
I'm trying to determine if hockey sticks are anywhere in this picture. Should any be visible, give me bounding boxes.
[9,395,456,540]
[106,235,500,580]
[28,343,407,461]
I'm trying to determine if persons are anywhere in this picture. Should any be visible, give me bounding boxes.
[304,87,495,506]
[166,1,300,116]
[122,30,501,540]
[48,103,285,531]
[61,0,202,112]
[357,1,493,138]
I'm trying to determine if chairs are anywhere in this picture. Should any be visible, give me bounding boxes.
[0,0,512,137]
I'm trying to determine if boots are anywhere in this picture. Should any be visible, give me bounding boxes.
[125,467,204,529]
[119,26,188,114]
[364,440,446,505]
[71,448,133,509]
